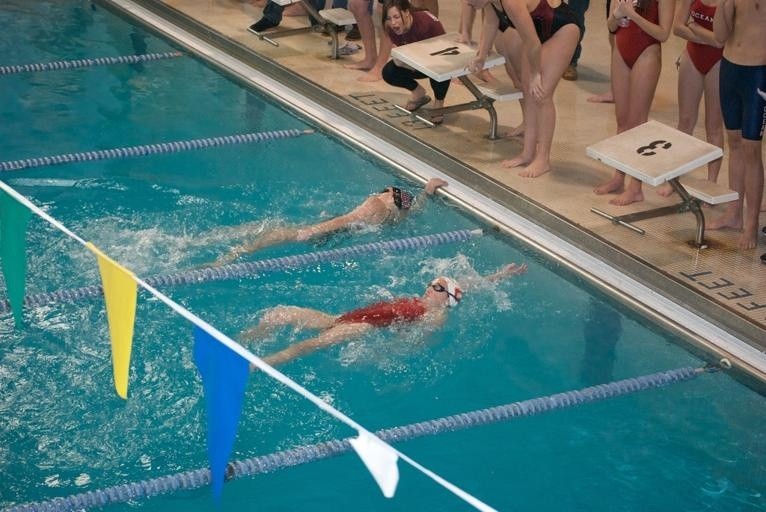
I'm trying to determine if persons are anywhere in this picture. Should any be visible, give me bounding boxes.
[231,261,528,370]
[250,0,451,125]
[454,0,590,177]
[588,0,765,248]
[189,175,449,272]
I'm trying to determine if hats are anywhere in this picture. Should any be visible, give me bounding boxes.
[392,184,412,210]
[443,276,461,308]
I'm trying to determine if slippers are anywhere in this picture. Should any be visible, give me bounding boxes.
[432,116,442,125]
[406,95,431,112]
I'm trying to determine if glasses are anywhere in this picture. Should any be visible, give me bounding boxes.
[381,188,391,195]
[428,282,458,300]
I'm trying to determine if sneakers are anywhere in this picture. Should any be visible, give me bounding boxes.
[249,18,273,32]
[322,25,345,36]
[345,28,360,40]
